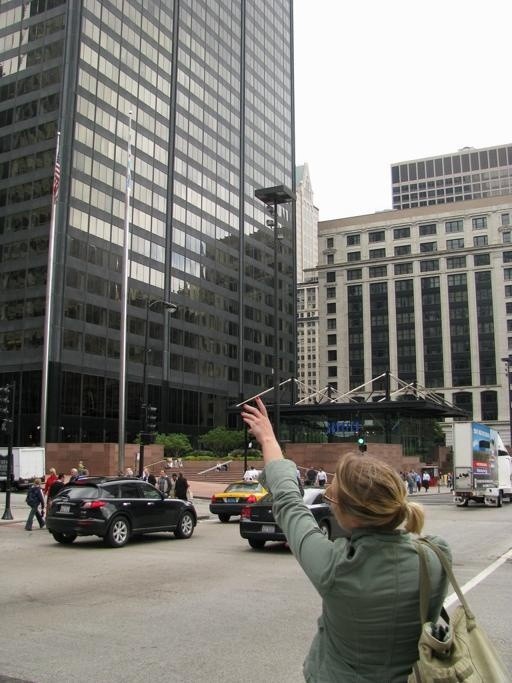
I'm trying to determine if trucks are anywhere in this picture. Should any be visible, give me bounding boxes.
[0,447,46,491]
[452,420,512,508]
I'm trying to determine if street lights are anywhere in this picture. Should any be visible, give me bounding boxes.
[253,184,295,446]
[138,299,178,479]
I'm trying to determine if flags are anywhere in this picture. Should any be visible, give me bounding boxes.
[50,133,63,206]
[124,123,138,199]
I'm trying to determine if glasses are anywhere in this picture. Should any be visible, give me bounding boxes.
[321,487,340,506]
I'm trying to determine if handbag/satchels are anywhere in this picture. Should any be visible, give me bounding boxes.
[404,536,507,683]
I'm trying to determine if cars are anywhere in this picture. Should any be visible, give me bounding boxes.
[239,488,352,550]
[209,469,270,524]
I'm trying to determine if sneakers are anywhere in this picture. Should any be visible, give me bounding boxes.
[23,527,33,531]
[39,523,45,529]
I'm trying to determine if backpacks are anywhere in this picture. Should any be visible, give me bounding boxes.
[26,488,40,507]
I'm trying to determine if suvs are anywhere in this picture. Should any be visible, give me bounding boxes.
[45,474,197,549]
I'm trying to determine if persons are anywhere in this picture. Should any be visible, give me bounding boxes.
[239,391,452,682]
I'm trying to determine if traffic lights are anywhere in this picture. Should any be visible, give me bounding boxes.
[141,433,156,444]
[1,419,14,436]
[141,404,159,432]
[358,435,364,446]
[0,388,12,417]
